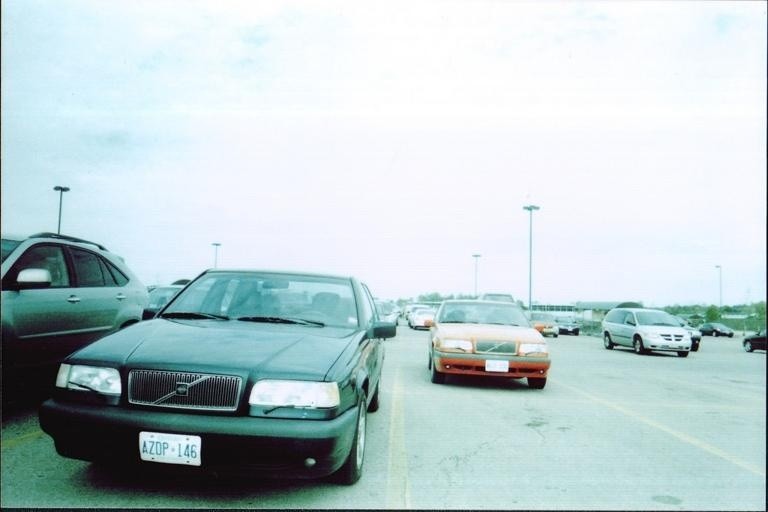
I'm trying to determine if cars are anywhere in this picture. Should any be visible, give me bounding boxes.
[601,308,767,356]
[405,294,580,389]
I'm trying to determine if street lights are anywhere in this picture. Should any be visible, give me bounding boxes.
[213,243,221,266]
[473,254,478,294]
[523,205,541,307]
[54,185,70,235]
